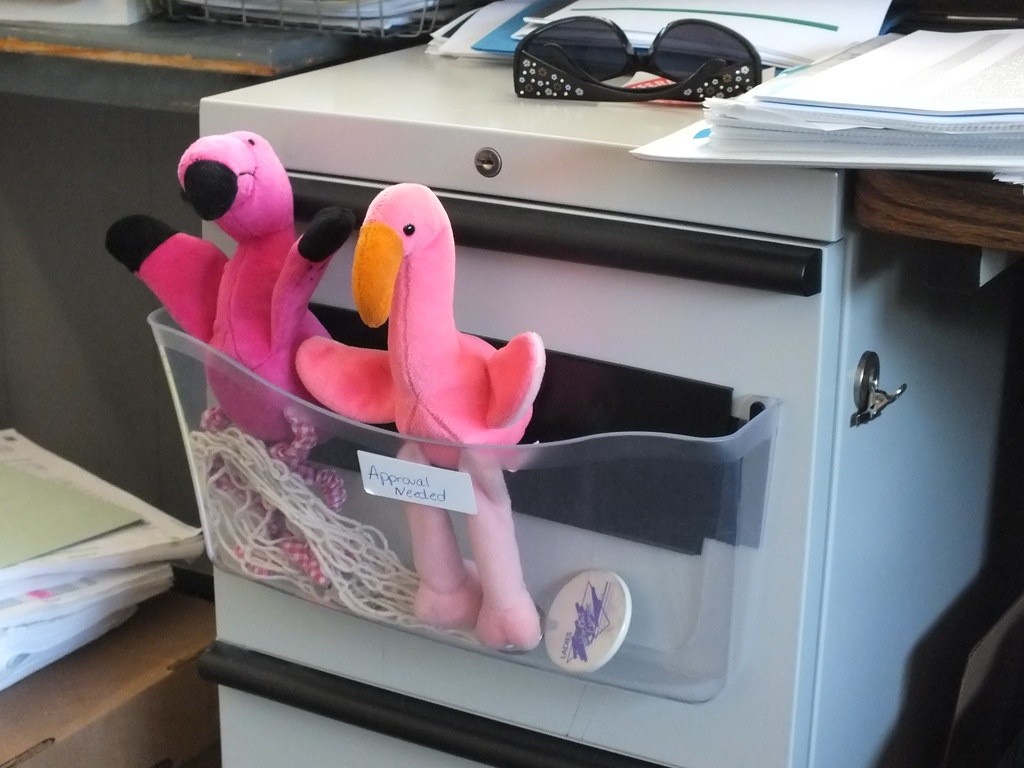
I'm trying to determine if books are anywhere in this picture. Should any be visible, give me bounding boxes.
[627,29,1024,171]
[0,427,208,692]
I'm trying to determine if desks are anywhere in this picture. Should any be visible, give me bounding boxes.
[0,11,441,115]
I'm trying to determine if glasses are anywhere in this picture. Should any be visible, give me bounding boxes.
[512,15,762,104]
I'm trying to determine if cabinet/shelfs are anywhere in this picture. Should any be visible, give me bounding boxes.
[146,47,1013,768]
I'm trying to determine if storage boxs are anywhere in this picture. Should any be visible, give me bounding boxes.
[0,591,220,768]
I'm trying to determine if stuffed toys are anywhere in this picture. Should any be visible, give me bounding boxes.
[295,183,546,651]
[104,128,356,449]
